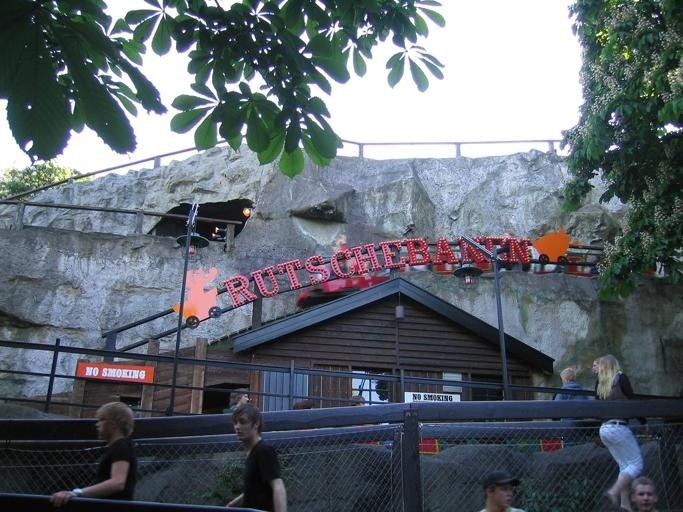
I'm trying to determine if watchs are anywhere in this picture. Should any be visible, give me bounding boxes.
[71,486,82,495]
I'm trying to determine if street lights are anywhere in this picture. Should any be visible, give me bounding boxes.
[453,231,513,419]
[162,201,212,412]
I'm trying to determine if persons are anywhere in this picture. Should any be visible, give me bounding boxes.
[554,368,592,446]
[228,387,252,412]
[627,475,660,512]
[596,355,648,511]
[222,403,287,511]
[293,399,312,412]
[478,468,524,511]
[348,395,378,445]
[46,401,135,511]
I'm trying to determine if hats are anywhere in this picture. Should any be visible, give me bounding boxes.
[483,471,520,489]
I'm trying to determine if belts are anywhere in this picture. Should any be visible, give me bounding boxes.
[605,421,626,426]
